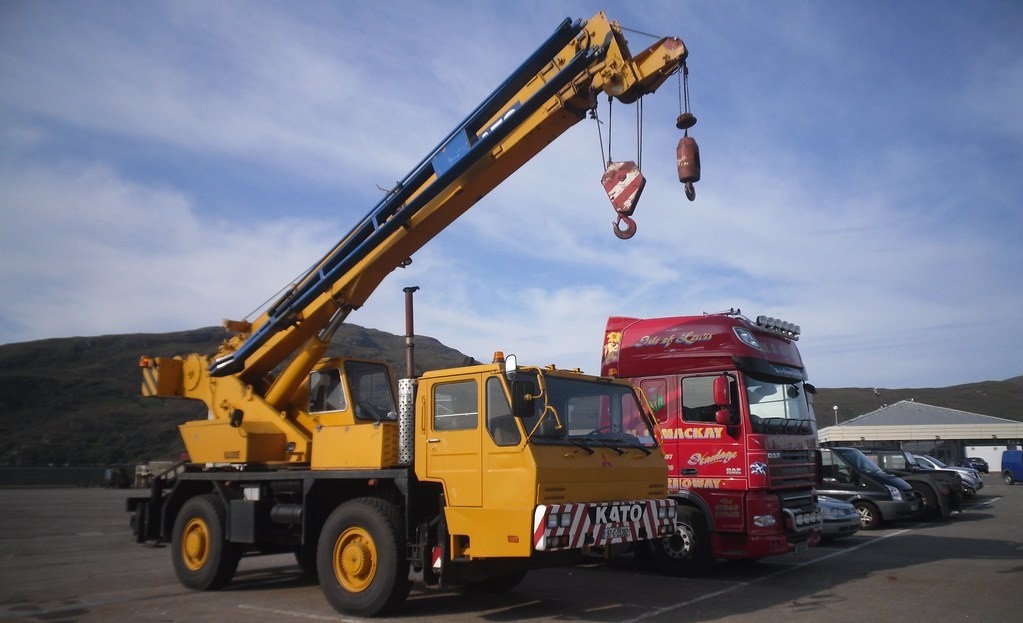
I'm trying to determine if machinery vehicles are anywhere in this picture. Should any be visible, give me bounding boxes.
[138,8,696,471]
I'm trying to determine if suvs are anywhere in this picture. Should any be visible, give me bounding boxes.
[860,450,963,520]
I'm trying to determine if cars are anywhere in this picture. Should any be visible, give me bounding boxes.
[879,455,983,499]
[959,458,989,475]
[815,496,862,538]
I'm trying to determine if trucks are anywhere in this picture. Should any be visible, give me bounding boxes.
[125,350,678,616]
[601,312,825,580]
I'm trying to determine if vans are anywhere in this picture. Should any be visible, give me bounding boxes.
[1001,450,1023,485]
[820,447,919,530]
